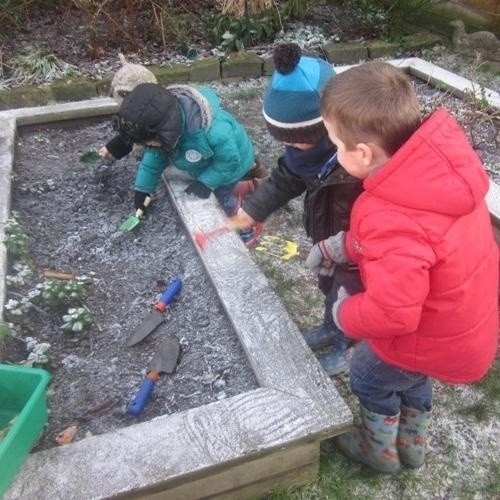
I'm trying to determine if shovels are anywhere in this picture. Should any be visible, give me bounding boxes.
[128,334,180,417]
[119,195,151,232]
[79,151,102,164]
[254,235,336,268]
[127,279,182,347]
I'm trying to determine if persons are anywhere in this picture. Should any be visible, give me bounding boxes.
[118,82,269,249]
[99,52,158,167]
[229,42,367,375]
[302,59,500,475]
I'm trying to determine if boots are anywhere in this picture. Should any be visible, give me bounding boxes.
[333,406,402,474]
[396,404,434,469]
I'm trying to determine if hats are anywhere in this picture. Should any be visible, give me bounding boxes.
[112,83,182,150]
[110,63,159,107]
[261,43,339,145]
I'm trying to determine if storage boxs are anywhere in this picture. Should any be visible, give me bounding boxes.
[1,364,54,498]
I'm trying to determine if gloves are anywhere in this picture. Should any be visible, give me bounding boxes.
[186,181,212,199]
[304,230,351,277]
[133,190,152,216]
[330,286,350,332]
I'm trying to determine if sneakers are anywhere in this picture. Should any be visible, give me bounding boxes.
[222,190,255,244]
[303,321,343,349]
[319,336,356,377]
